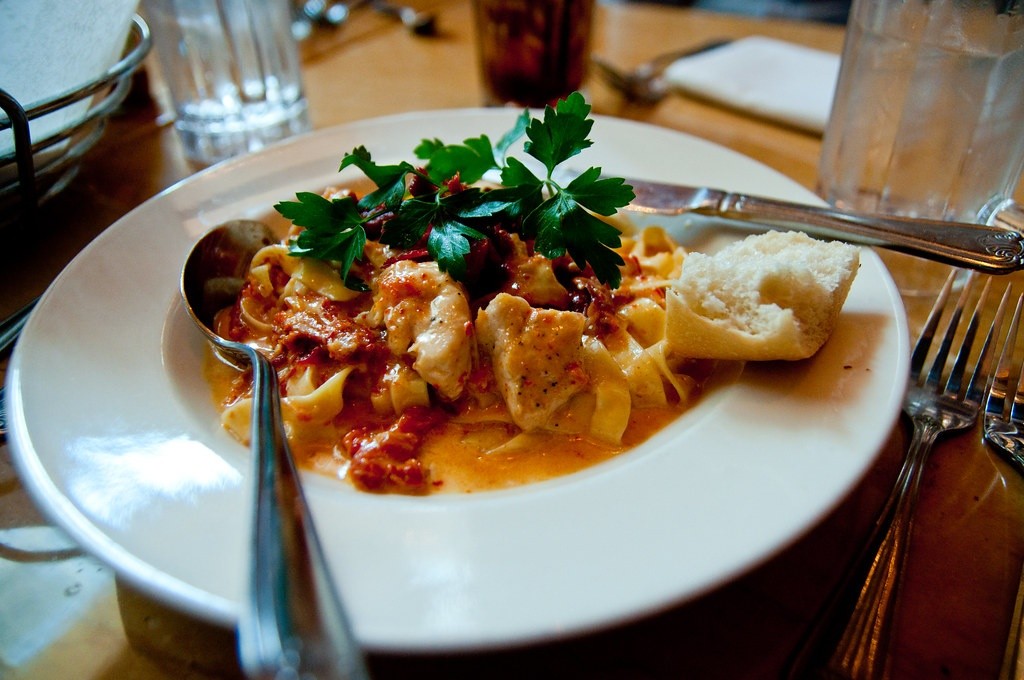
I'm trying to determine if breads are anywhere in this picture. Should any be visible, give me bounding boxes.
[664,228,859,360]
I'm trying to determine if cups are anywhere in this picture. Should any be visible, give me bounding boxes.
[144,0,310,164]
[472,0,596,104]
[820,0,1024,236]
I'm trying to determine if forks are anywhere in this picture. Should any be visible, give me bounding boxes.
[597,39,733,102]
[984,293,1024,680]
[776,269,1013,680]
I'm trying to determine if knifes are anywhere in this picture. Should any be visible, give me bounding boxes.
[565,173,1024,275]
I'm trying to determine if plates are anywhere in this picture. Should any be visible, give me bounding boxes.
[7,106,908,652]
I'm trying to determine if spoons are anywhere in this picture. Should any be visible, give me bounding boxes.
[183,221,369,680]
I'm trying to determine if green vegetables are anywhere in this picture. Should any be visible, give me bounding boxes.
[272,91,637,290]
